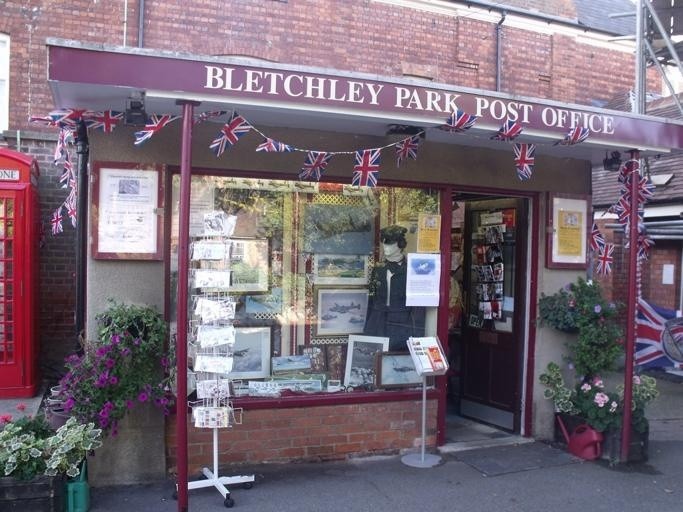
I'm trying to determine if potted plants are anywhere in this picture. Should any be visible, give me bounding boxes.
[0,417,101,511]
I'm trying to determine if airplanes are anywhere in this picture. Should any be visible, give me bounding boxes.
[321,302,364,324]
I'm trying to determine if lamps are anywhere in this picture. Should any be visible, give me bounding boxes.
[385,122,426,147]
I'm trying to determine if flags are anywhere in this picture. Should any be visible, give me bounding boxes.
[132,112,183,145]
[489,118,524,144]
[191,108,230,127]
[604,157,657,260]
[393,134,419,169]
[513,143,535,182]
[589,224,605,251]
[206,111,253,157]
[349,147,384,188]
[553,126,591,148]
[594,242,616,278]
[254,138,296,154]
[26,108,126,239]
[297,150,334,180]
[632,295,683,376]
[434,108,479,135]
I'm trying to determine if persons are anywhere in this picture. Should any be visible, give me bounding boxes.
[361,223,427,354]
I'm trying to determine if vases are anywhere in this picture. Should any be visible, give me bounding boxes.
[551,412,651,471]
[43,383,76,432]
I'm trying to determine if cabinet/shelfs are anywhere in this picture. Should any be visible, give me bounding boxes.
[170,208,258,508]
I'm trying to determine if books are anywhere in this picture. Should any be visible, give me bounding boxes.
[468,207,516,322]
[189,209,237,427]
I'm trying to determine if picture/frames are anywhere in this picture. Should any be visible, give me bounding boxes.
[545,190,594,272]
[86,160,167,263]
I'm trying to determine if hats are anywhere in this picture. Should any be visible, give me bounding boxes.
[380,225,408,245]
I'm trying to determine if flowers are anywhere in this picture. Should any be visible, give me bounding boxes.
[533,276,658,437]
[0,293,170,437]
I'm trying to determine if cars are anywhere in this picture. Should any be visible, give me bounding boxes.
[318,262,364,279]
